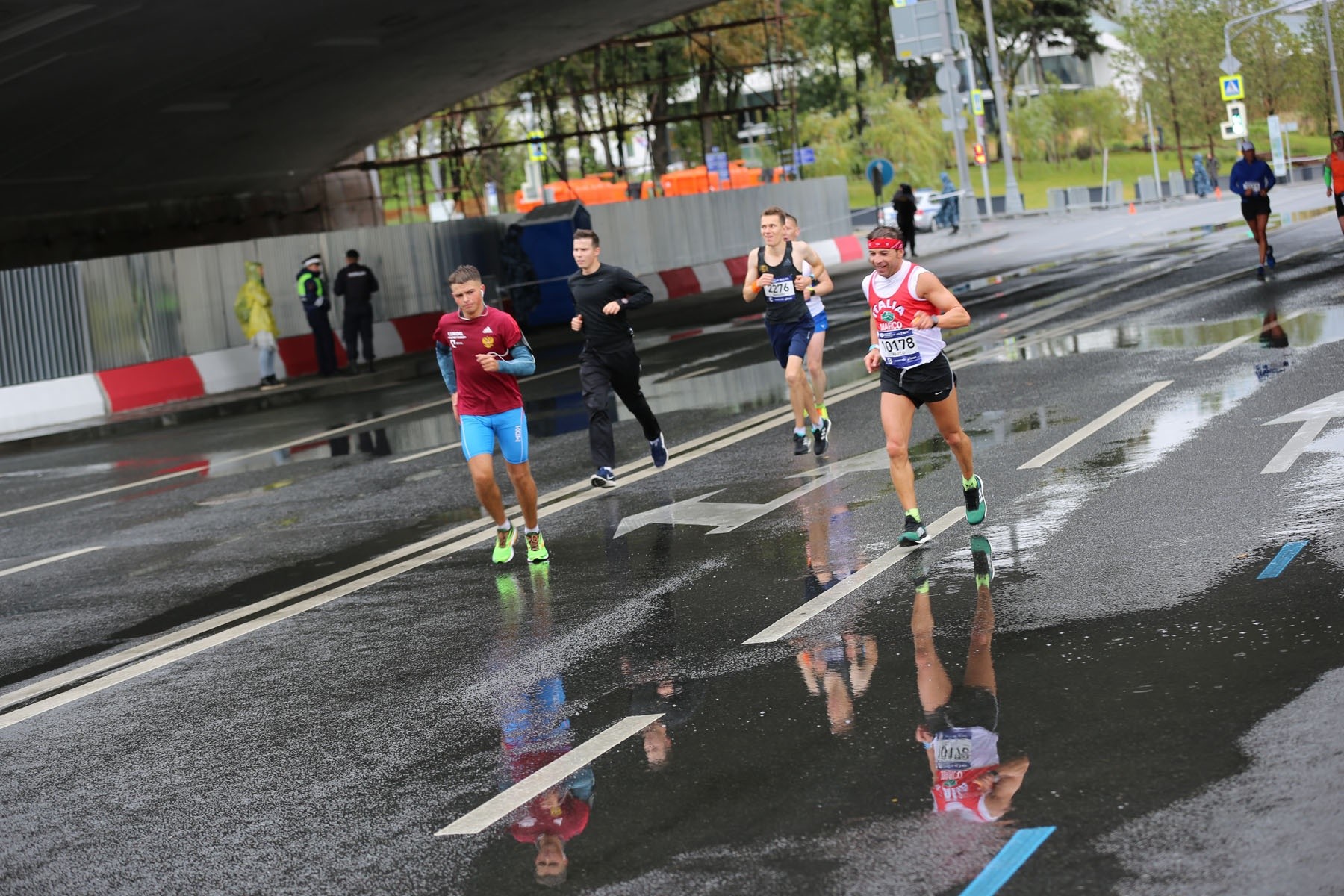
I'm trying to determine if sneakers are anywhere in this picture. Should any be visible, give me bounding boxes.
[591,467,617,488]
[492,519,518,564]
[811,417,831,456]
[898,515,926,544]
[525,530,549,562]
[794,431,812,454]
[963,474,988,524]
[970,536,995,582]
[648,431,668,469]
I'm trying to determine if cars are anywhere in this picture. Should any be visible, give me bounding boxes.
[878,188,944,232]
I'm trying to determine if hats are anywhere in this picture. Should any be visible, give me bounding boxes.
[1242,141,1254,152]
[301,254,323,268]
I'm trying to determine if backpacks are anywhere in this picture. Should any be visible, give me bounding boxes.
[236,297,254,323]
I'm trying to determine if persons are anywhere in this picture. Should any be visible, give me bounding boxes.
[908,542,1030,825]
[1255,312,1291,382]
[495,225,539,337]
[1324,130,1344,234]
[233,260,287,390]
[333,248,379,374]
[862,225,988,548]
[792,477,879,734]
[892,183,918,258]
[936,174,959,235]
[1229,142,1276,280]
[567,228,669,489]
[1193,152,1220,198]
[742,207,831,457]
[295,254,346,376]
[432,265,549,564]
[602,496,681,770]
[782,212,835,436]
[492,561,595,890]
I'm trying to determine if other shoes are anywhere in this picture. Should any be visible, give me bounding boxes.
[806,404,830,420]
[261,375,286,391]
[1266,246,1275,267]
[952,225,959,233]
[1258,270,1265,279]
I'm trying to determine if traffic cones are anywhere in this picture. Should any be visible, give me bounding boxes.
[1214,187,1221,196]
[1128,203,1137,215]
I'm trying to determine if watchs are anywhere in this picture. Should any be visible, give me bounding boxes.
[620,298,629,310]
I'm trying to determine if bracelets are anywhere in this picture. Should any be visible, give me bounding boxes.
[868,344,880,352]
[808,274,816,284]
[751,279,761,293]
[990,770,1000,783]
[808,287,815,296]
[930,314,938,329]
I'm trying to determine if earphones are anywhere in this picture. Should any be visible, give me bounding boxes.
[480,289,484,297]
